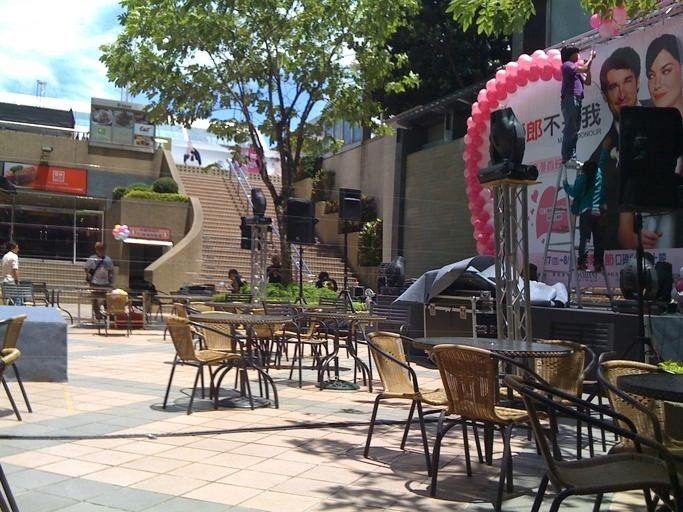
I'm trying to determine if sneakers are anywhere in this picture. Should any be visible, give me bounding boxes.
[564,158,583,167]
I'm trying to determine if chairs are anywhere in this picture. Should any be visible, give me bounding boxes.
[428,343,562,511]
[1,465,20,511]
[599,360,683,512]
[364,330,483,476]
[517,339,606,459]
[504,374,682,511]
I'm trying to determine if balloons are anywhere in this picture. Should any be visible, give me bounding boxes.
[461,48,563,261]
[587,4,628,39]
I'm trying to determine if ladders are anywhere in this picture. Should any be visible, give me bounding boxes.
[539,163,616,310]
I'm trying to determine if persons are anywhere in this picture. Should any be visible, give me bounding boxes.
[1,240,21,307]
[266,253,284,284]
[225,269,247,293]
[643,252,659,302]
[572,47,642,268]
[84,242,114,319]
[521,263,538,282]
[315,273,338,291]
[617,35,682,249]
[560,45,596,169]
[75,133,90,142]
[562,158,612,274]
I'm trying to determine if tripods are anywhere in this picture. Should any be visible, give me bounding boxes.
[577,212,675,413]
[320,223,359,314]
[293,244,308,304]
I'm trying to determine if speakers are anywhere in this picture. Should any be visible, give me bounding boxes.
[488,107,525,165]
[286,197,313,215]
[286,214,314,244]
[339,187,362,219]
[618,105,683,204]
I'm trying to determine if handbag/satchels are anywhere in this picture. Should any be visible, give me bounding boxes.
[86,272,92,281]
